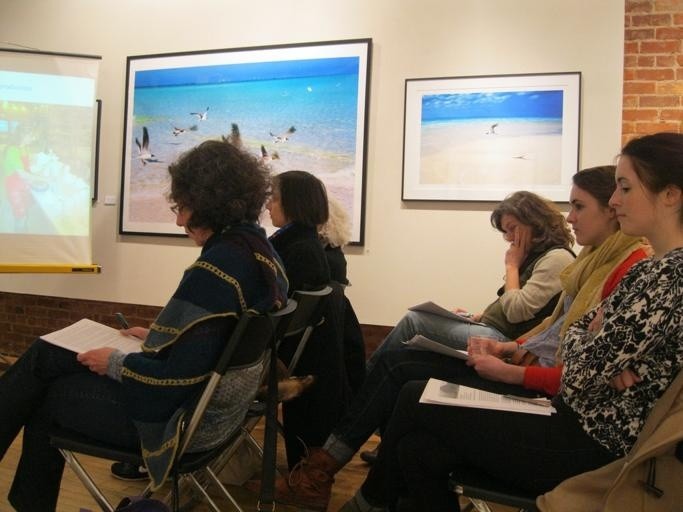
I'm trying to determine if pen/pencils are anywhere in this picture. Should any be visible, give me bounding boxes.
[505,395,551,406]
[116,312,130,330]
[458,313,471,316]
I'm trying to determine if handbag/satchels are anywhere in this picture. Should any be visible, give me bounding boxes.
[114,495,170,512]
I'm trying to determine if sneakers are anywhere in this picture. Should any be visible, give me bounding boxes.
[111,461,172,483]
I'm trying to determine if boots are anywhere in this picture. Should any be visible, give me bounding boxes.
[243,436,344,511]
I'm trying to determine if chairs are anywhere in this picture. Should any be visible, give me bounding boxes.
[50,298,293,512]
[195,278,351,499]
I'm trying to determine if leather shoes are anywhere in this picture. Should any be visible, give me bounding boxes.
[360,442,379,463]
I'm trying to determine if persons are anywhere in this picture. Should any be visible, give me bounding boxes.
[316,193,352,344]
[265,170,332,375]
[337,132,682,512]
[241,165,654,511]
[0,138,292,512]
[363,190,575,380]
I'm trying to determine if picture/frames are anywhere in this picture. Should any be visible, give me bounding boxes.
[119,33,372,243]
[400,70,580,205]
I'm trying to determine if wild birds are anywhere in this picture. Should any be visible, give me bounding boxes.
[130,106,298,169]
[484,123,498,134]
